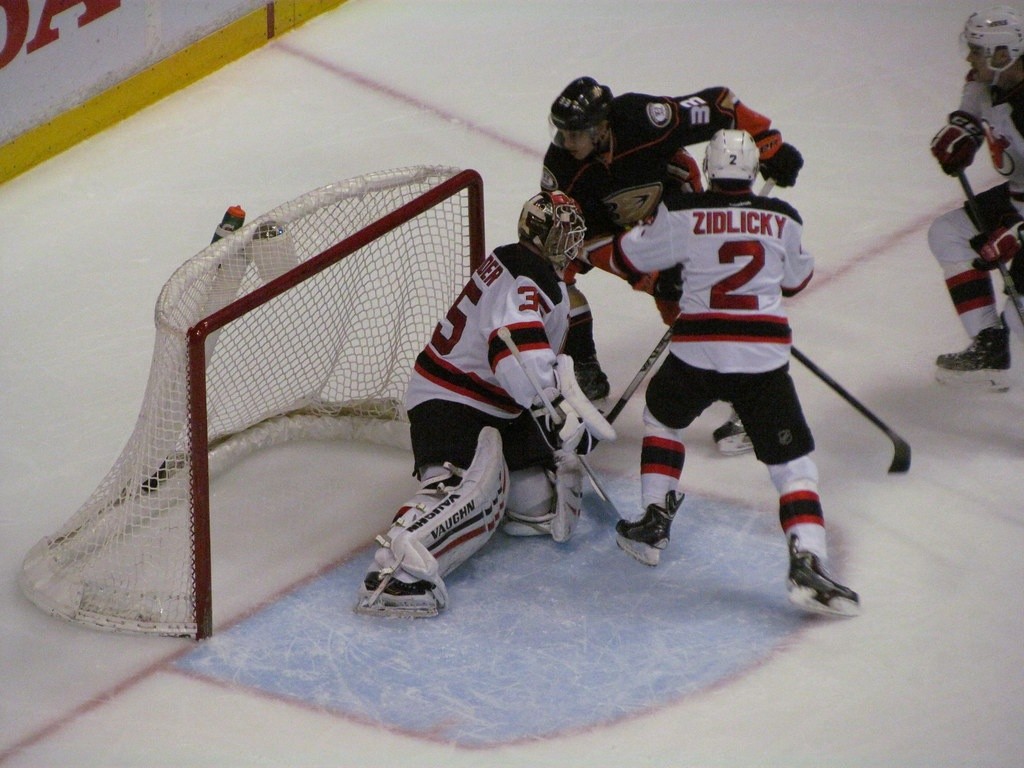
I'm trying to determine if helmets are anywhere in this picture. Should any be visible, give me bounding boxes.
[518,190,587,281]
[703,129,760,180]
[964,5,1024,60]
[551,76,613,131]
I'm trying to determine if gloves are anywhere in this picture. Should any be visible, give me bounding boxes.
[754,130,804,188]
[930,109,987,177]
[969,202,1024,272]
[628,263,683,300]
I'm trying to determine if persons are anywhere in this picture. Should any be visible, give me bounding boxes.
[354,76,856,617]
[928,8,1024,392]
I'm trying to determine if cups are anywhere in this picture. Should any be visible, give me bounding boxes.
[211,204,246,269]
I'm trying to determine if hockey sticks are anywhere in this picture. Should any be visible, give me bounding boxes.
[957,170,1023,327]
[497,314,684,525]
[788,345,912,474]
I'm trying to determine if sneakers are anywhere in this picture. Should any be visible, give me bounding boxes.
[786,534,858,618]
[934,311,1011,390]
[355,571,442,620]
[615,490,685,567]
[712,404,754,456]
[573,353,610,402]
